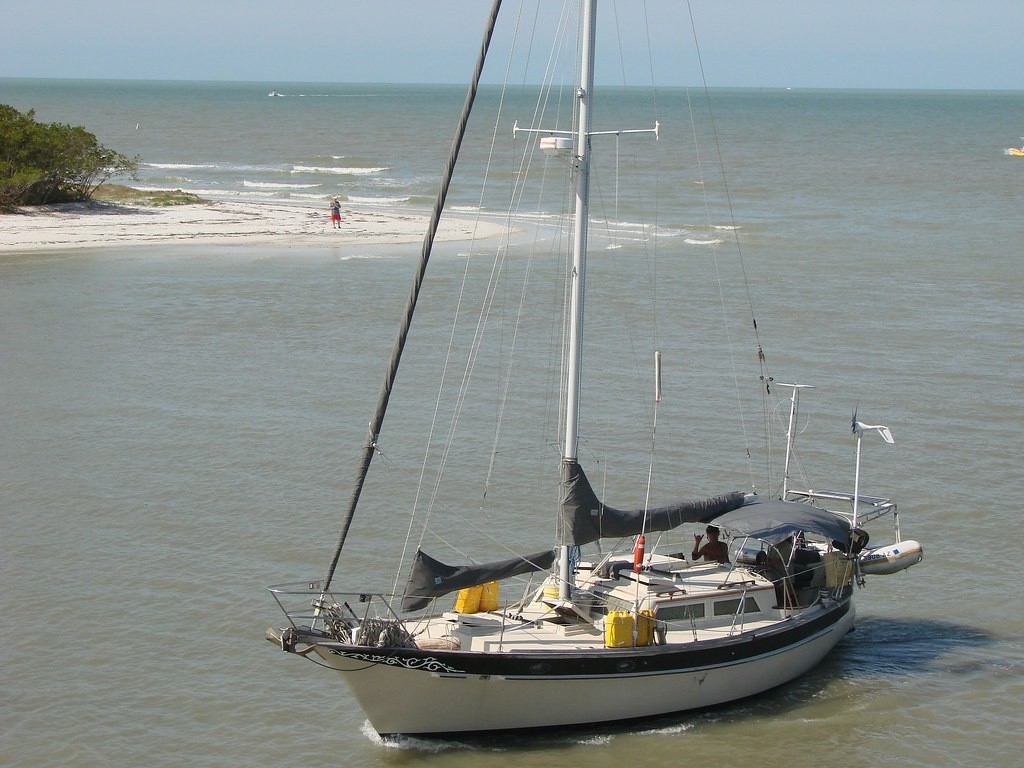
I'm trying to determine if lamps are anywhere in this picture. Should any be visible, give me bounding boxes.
[308,581,319,589]
[359,593,372,603]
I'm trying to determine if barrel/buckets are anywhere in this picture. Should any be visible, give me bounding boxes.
[604,609,655,647]
[455,582,500,614]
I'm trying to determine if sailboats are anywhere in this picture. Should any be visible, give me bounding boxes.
[264,0,925,740]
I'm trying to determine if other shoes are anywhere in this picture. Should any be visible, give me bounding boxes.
[334,227,336,228]
[338,226,341,228]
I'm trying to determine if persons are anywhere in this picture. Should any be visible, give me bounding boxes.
[692,526,731,564]
[768,537,793,607]
[330,197,342,229]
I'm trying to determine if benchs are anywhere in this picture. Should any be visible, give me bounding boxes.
[793,548,827,589]
